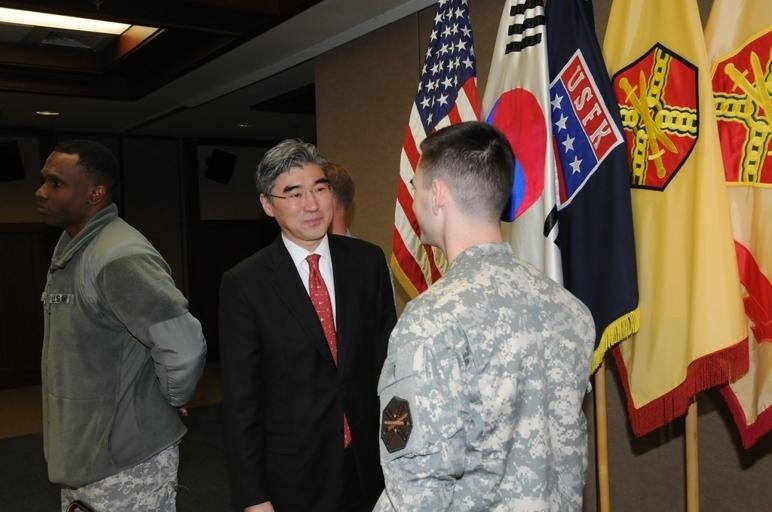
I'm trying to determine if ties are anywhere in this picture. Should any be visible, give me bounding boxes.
[306,255,352,450]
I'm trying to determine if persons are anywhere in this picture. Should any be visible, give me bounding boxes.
[36,140,207,512]
[373,120,596,512]
[325,163,396,307]
[219,139,397,512]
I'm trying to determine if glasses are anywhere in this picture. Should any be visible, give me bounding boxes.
[268,184,332,200]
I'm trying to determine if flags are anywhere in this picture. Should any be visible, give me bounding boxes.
[389,0,481,323]
[601,0,749,439]
[703,0,772,448]
[545,0,641,375]
[482,0,564,287]
